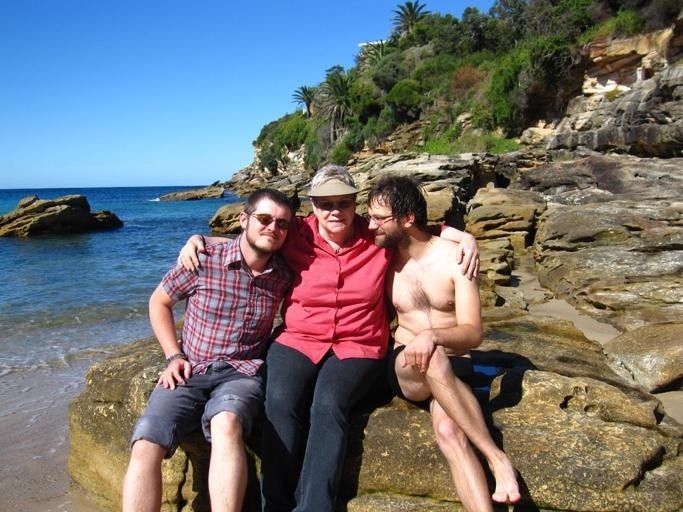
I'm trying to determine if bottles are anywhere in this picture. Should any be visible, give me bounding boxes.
[306,179,360,197]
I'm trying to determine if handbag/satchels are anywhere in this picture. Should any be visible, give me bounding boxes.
[200,234,206,244]
[165,353,186,366]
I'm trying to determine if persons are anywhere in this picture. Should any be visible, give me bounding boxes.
[121,188,296,512]
[178,166,480,512]
[368,175,522,512]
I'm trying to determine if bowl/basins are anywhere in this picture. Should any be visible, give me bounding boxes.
[326,229,351,253]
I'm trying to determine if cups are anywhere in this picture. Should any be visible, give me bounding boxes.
[251,214,288,230]
[315,200,352,209]
[368,215,398,222]
